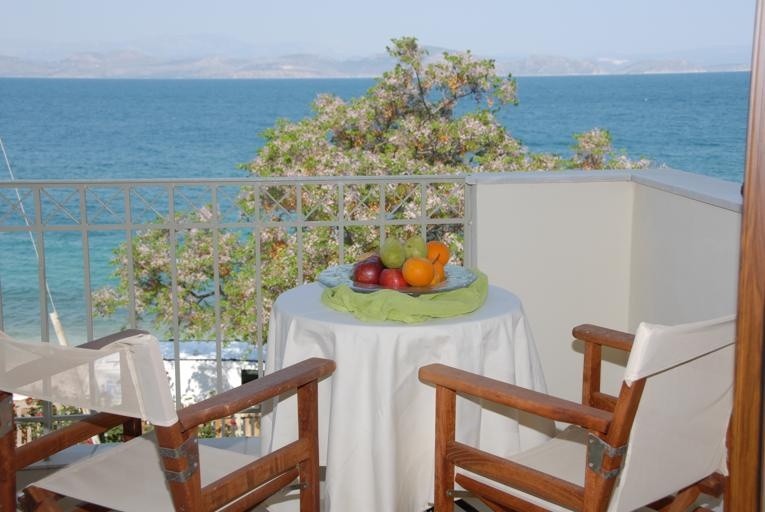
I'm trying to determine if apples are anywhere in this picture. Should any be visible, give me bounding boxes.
[366,256,383,271]
[353,261,380,284]
[378,269,408,289]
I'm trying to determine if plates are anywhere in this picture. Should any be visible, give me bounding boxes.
[316,261,481,297]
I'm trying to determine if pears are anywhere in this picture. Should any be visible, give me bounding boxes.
[379,236,404,269]
[403,233,427,259]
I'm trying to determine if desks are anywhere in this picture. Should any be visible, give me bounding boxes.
[264,277,562,512]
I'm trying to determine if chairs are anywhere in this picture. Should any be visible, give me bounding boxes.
[416,310,737,512]
[0,325,341,512]
